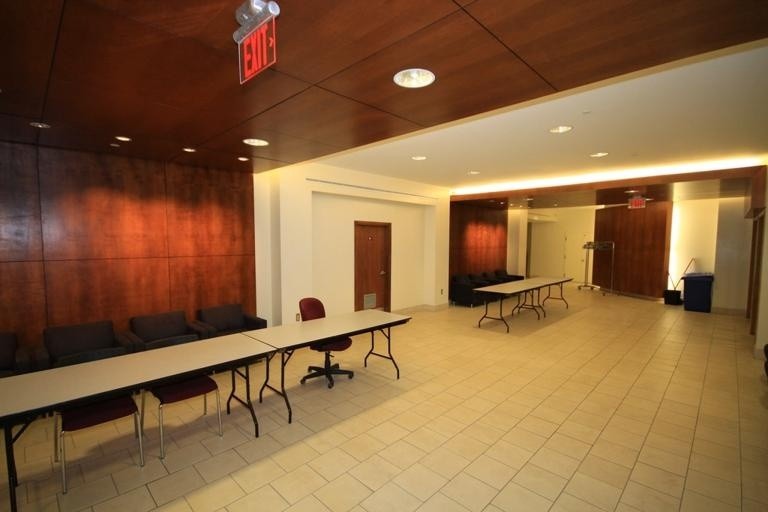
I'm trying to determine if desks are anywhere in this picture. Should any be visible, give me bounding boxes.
[241,308,412,424]
[0,309,276,512]
[472,277,574,333]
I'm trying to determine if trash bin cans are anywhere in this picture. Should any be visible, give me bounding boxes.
[681,273,713,313]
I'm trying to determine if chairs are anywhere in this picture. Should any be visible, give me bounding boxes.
[451,269,524,308]
[299,298,354,388]
[41,320,145,495]
[124,309,223,459]
[192,303,267,339]
